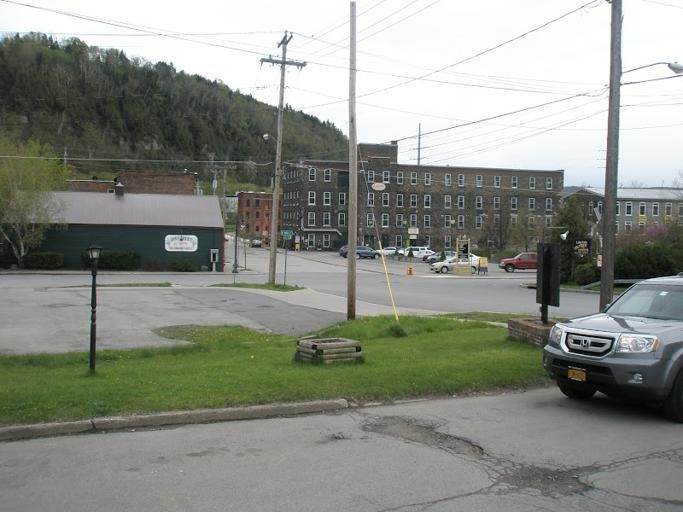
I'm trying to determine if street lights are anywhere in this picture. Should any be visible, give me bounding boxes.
[260,133,282,285]
[596,59,683,315]
[83,241,103,381]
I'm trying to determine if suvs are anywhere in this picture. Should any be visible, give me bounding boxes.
[541,270,683,420]
[499,250,537,272]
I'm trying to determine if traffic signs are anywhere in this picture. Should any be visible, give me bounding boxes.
[280,230,296,236]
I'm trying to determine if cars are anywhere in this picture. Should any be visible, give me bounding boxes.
[247,237,262,248]
[338,243,485,274]
